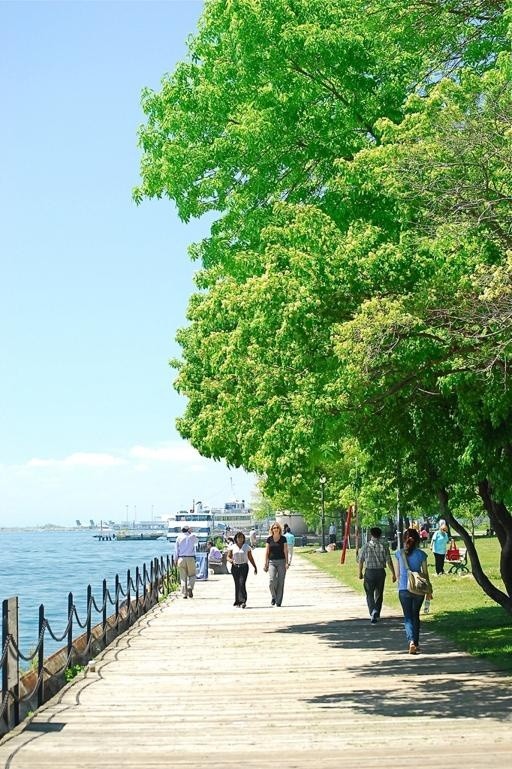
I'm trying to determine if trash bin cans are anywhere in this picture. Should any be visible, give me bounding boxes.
[195,552,208,581]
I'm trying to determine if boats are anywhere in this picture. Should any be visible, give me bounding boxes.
[166,500,256,542]
[117,529,165,541]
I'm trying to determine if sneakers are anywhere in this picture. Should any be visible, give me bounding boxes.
[371,610,380,623]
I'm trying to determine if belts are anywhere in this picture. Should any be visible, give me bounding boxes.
[178,556,194,557]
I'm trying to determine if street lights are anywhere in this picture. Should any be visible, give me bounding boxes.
[319,473,329,553]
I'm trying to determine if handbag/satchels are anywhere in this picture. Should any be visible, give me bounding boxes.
[447,541,459,560]
[407,570,433,596]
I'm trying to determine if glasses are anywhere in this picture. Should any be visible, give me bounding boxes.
[273,527,279,529]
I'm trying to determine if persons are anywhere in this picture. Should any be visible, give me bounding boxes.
[358,527,397,623]
[330,521,337,543]
[206,540,222,562]
[420,527,430,548]
[395,528,433,654]
[486,528,494,537]
[226,532,258,608]
[430,524,455,576]
[175,524,196,599]
[222,524,233,546]
[263,522,289,607]
[283,523,295,566]
[249,528,257,549]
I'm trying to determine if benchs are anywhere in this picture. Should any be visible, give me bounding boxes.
[208,552,229,574]
[443,549,470,575]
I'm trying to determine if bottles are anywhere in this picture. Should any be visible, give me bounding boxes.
[424,599,430,613]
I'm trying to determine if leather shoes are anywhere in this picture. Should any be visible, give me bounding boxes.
[184,588,193,599]
[271,599,281,607]
[409,644,416,654]
